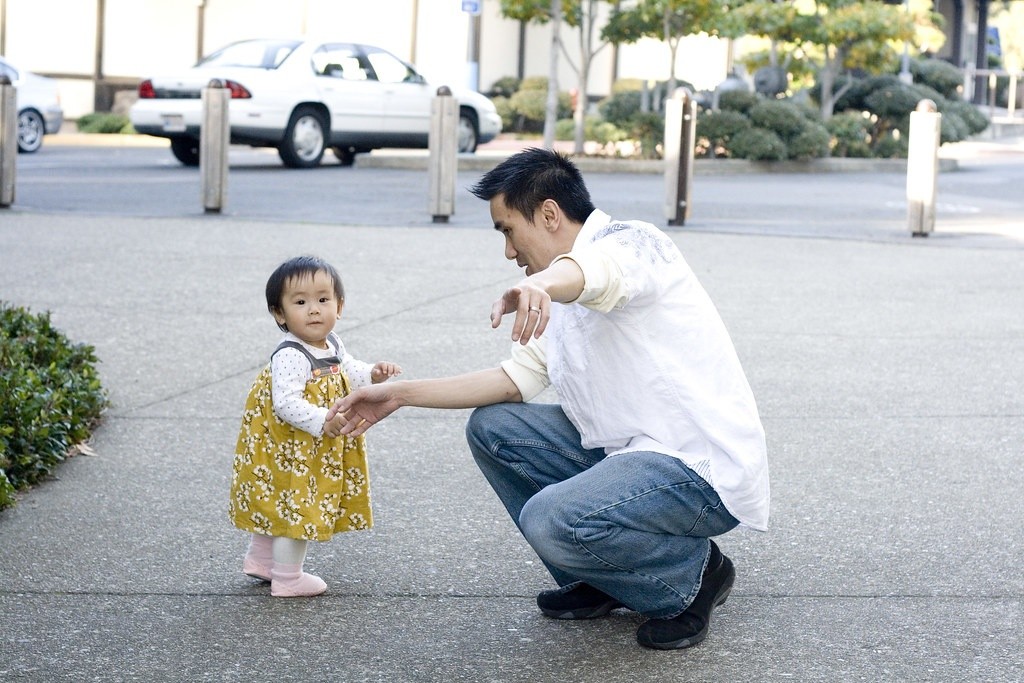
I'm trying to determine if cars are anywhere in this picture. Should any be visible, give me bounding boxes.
[128,36,504,170]
[0,55,64,155]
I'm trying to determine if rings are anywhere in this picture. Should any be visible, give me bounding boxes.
[529,306,537,311]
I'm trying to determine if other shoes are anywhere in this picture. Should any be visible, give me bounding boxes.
[244,558,274,580]
[270,573,327,597]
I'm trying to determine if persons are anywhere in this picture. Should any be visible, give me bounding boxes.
[326,147,770,651]
[229,255,403,598]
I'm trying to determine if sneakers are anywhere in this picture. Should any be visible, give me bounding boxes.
[637,554,735,649]
[537,581,634,619]
